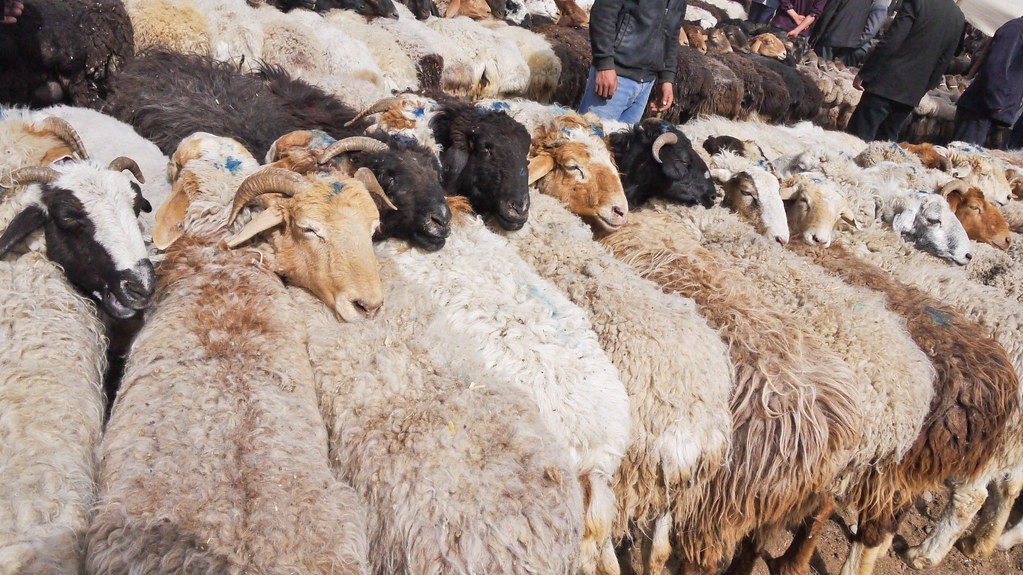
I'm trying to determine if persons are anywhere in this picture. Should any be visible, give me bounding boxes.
[750,0,780,25]
[848,0,965,144]
[772,0,825,43]
[580,0,689,123]
[809,0,892,70]
[951,15,1023,147]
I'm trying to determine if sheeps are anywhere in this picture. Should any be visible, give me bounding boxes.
[0,0,1023,575]
